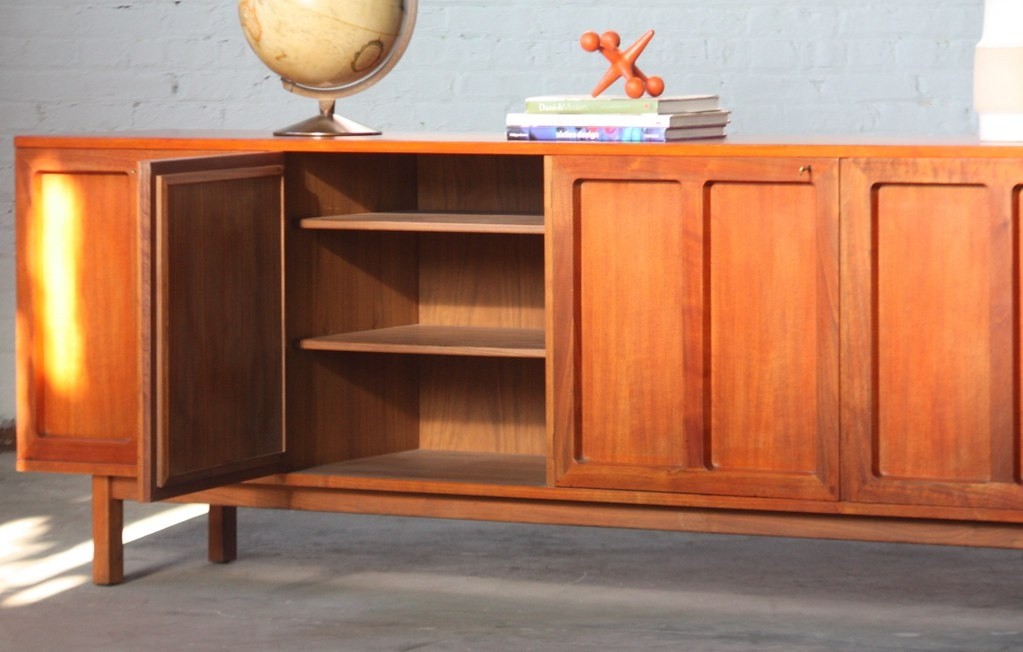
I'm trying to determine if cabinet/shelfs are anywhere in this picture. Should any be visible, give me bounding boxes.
[11,134,1023,587]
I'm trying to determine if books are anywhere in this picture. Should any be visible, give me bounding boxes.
[506,94,731,141]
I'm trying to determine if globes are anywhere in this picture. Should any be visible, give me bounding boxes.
[236,0,419,138]
